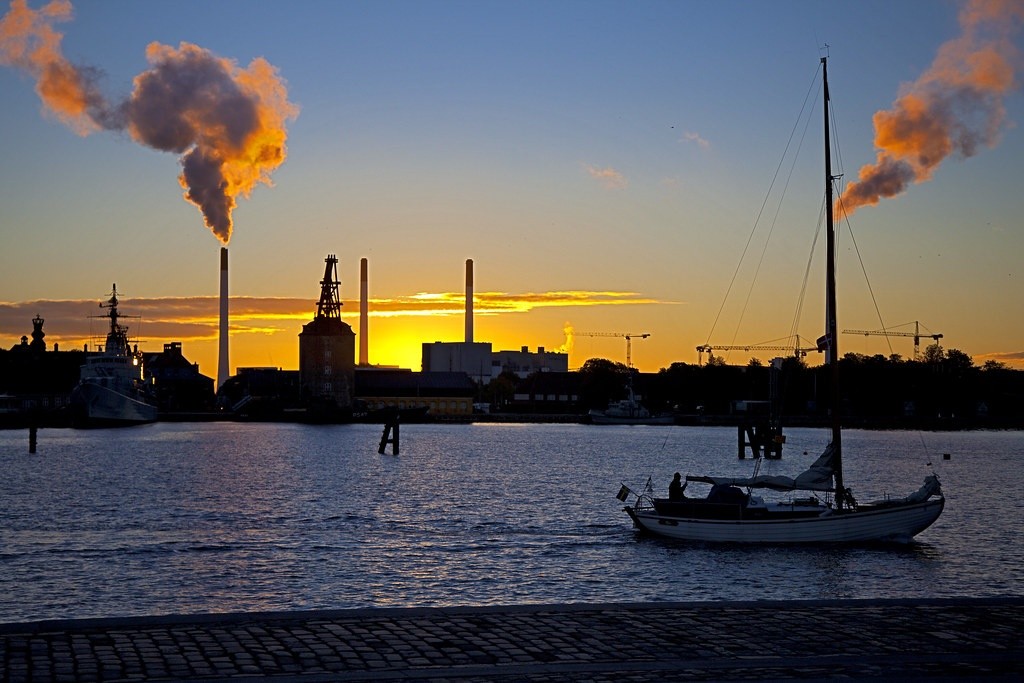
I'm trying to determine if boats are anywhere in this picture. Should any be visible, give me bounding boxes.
[589,376,682,425]
[72,284,161,429]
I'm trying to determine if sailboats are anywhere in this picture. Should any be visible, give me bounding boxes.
[617,55,946,543]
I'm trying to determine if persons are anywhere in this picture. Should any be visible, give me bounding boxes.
[668,472,688,500]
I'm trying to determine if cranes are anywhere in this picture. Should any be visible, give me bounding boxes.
[557,332,650,371]
[696,334,818,363]
[842,321,943,361]
